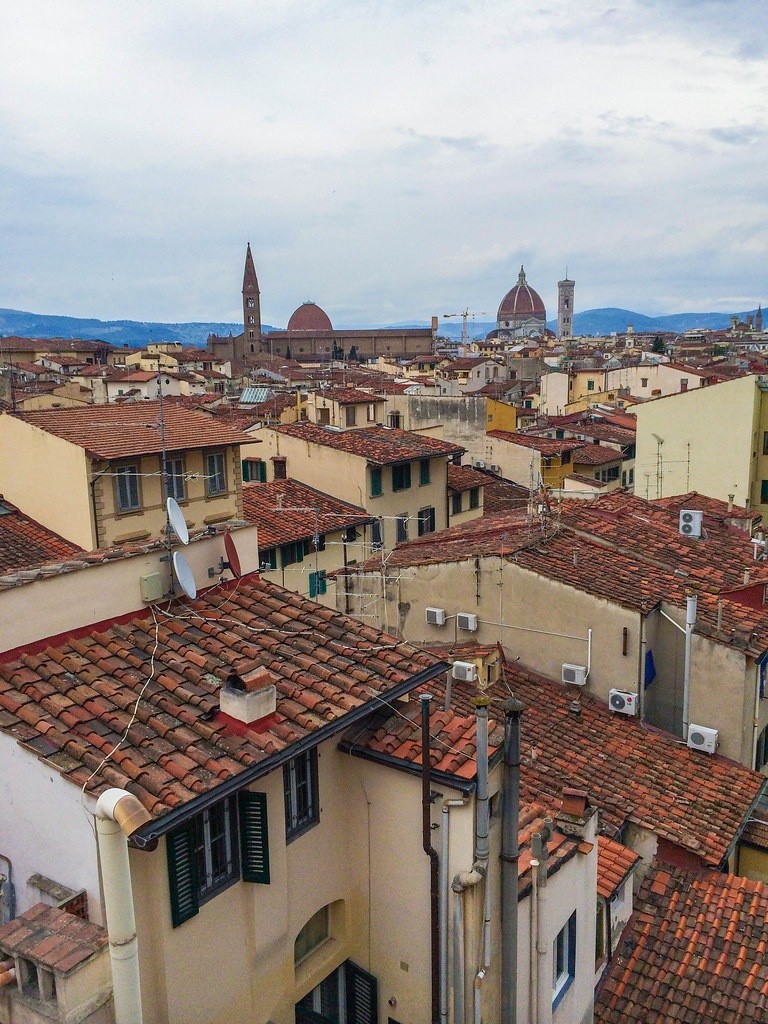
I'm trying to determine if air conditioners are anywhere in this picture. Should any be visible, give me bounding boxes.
[425,607,445,626]
[686,723,719,755]
[457,612,478,632]
[680,510,704,537]
[476,461,484,468]
[577,435,584,440]
[452,662,476,682]
[609,688,639,715]
[562,663,586,685]
[491,464,499,471]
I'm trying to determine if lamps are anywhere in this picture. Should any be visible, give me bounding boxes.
[261,562,271,572]
